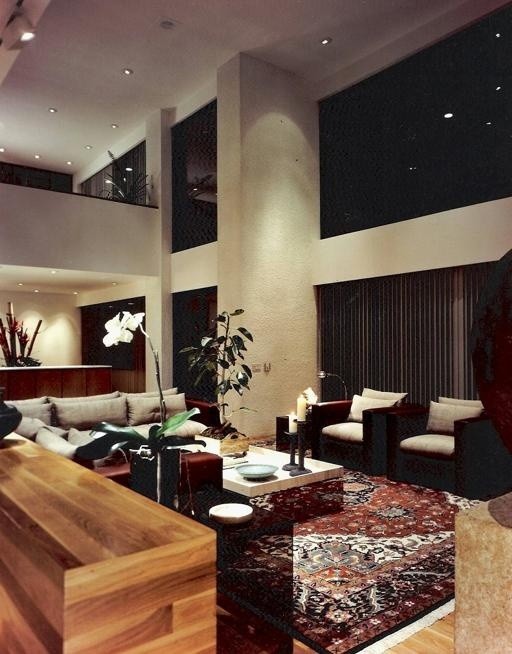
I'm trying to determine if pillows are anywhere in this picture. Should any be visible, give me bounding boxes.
[5,387,209,461]
[347,388,483,434]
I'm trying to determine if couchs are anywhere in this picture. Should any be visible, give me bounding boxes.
[89,451,223,535]
[0,398,222,437]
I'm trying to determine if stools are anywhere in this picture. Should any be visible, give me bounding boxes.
[208,501,256,583]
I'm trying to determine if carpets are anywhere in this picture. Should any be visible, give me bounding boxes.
[245,435,484,654]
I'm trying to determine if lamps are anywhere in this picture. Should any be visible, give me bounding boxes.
[317,370,348,400]
[12,14,36,40]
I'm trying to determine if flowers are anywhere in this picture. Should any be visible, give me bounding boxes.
[72,311,206,461]
[0,318,29,367]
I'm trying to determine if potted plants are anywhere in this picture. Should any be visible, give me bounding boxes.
[179,308,253,456]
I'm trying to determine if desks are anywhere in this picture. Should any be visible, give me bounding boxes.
[0,430,218,654]
[453,491,512,654]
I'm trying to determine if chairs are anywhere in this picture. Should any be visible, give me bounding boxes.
[388,396,512,501]
[312,387,420,476]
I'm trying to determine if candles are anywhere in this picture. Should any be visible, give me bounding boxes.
[289,412,298,433]
[297,394,307,421]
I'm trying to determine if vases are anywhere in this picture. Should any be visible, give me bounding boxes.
[129,449,180,511]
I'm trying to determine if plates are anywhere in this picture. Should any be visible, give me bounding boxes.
[237,465,279,478]
